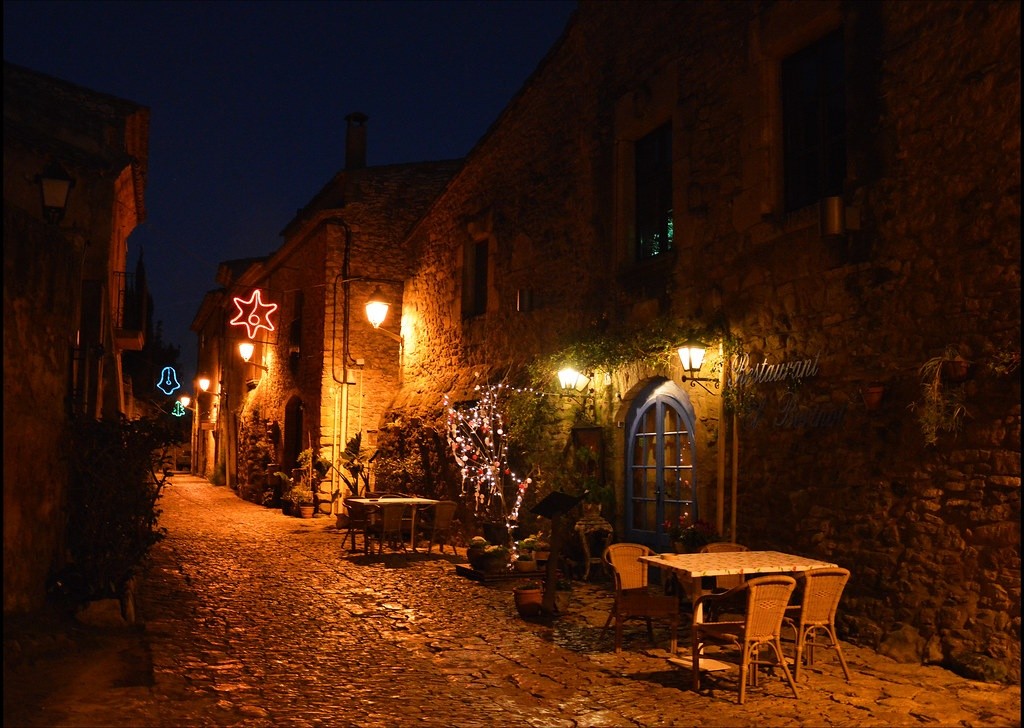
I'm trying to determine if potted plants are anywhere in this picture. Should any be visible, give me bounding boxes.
[280,491,315,518]
[581,484,604,518]
[906,343,1021,449]
[466,540,551,616]
[856,379,890,411]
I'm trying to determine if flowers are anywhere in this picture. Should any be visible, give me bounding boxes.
[661,515,721,547]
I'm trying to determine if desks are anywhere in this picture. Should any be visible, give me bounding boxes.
[638,551,838,671]
[348,498,439,549]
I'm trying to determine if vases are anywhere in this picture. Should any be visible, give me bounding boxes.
[482,520,509,544]
[674,542,689,553]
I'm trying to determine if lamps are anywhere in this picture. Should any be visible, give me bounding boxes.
[181,392,196,413]
[673,328,720,397]
[365,285,404,348]
[818,196,845,240]
[239,339,268,372]
[517,288,532,312]
[34,157,77,226]
[558,365,596,417]
[198,371,220,398]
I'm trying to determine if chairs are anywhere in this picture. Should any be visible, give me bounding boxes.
[341,495,457,558]
[599,543,851,705]
